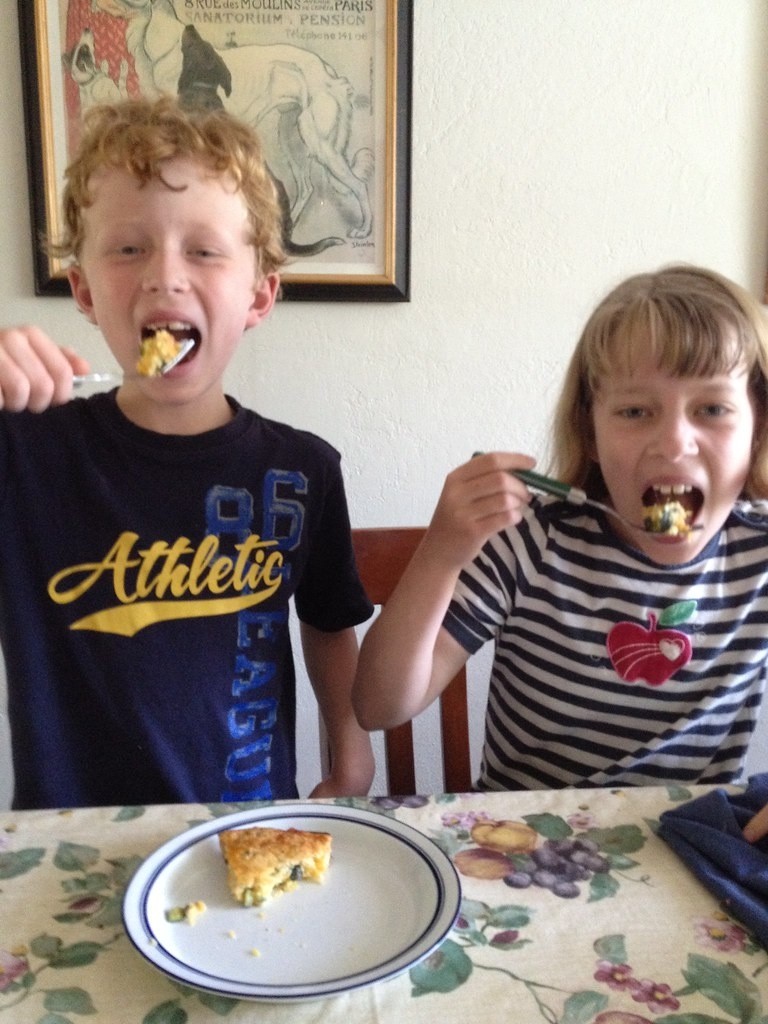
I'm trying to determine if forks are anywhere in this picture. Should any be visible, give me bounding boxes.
[471,452,704,534]
[70,338,195,384]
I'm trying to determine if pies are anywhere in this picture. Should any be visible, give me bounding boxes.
[218,830,332,907]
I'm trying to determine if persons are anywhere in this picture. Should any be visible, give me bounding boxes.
[0,94,377,815]
[352,262,768,841]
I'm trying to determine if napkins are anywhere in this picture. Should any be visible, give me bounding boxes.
[656,772,768,955]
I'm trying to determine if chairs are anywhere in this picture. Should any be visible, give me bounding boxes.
[318,528,472,795]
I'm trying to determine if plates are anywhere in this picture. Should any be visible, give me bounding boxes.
[121,804,463,1004]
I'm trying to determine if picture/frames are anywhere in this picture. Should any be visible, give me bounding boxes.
[16,0,414,303]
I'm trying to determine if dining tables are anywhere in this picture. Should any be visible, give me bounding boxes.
[0,785,768,1024]
